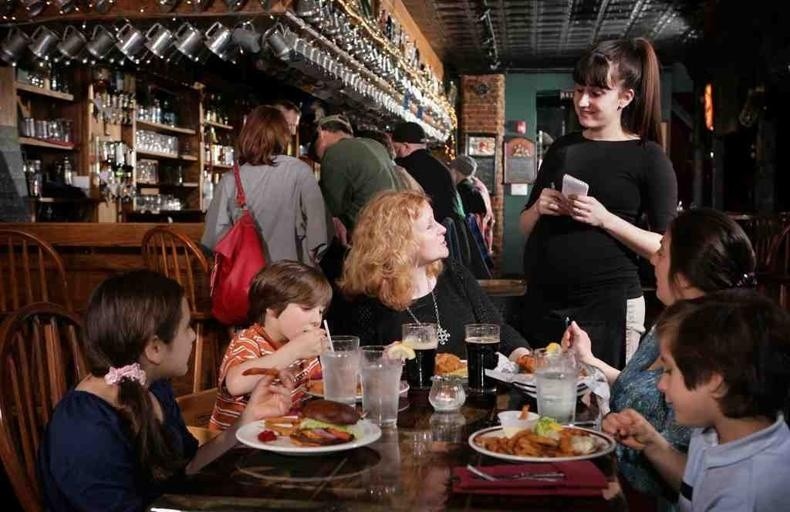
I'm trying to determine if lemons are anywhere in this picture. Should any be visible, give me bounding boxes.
[386,342,417,361]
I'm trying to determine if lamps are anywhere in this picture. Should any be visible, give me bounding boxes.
[477,6,502,71]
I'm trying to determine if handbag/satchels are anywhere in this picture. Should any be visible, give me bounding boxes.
[209,210,266,326]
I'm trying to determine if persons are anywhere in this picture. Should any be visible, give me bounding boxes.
[270,99,302,137]
[601,287,790,512]
[202,105,327,267]
[517,36,679,370]
[328,190,533,381]
[38,268,295,509]
[309,99,496,276]
[208,260,334,428]
[562,206,756,500]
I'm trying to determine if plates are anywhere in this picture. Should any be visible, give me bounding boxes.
[303,380,410,403]
[235,415,383,457]
[468,425,617,464]
[234,447,382,483]
[356,396,410,412]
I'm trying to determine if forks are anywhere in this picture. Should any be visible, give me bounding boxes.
[466,462,566,482]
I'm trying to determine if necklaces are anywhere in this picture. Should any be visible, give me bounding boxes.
[406,289,451,346]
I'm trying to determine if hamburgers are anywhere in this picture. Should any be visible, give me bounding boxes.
[290,401,361,447]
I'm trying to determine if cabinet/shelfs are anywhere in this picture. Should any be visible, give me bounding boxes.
[203,94,234,170]
[81,67,136,201]
[15,80,81,203]
[136,78,199,212]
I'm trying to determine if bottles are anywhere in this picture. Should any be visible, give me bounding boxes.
[344,0,444,97]
[428,412,467,443]
[15,54,236,216]
[428,375,466,412]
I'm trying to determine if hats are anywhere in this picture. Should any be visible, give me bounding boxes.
[445,155,478,177]
[308,113,353,164]
[392,122,426,143]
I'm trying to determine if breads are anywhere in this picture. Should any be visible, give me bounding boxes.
[434,353,465,375]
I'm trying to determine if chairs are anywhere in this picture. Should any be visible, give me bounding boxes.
[0,301,89,512]
[739,211,790,273]
[0,228,69,315]
[141,225,218,393]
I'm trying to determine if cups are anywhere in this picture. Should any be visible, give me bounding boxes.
[464,322,501,394]
[318,334,360,408]
[0,0,452,149]
[402,321,439,392]
[533,347,578,427]
[357,344,404,428]
[497,410,540,438]
